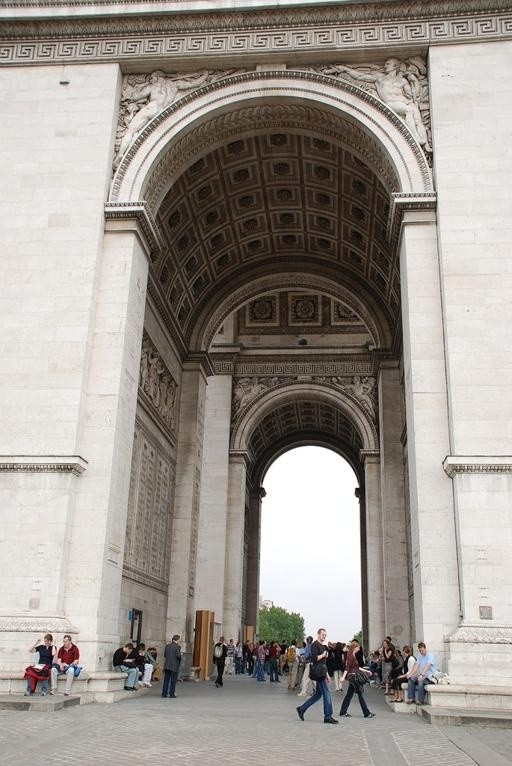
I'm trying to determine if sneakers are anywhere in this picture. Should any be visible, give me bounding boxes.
[324,717,338,724]
[50,690,57,695]
[366,713,376,718]
[41,691,47,696]
[133,687,138,690]
[296,707,304,721]
[65,692,69,696]
[340,713,351,717]
[124,686,133,691]
[162,694,167,698]
[170,694,176,698]
[25,691,32,696]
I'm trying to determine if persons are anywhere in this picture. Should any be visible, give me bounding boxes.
[49,635,79,695]
[23,634,57,695]
[114,643,157,691]
[229,373,380,429]
[139,333,180,431]
[213,628,436,723]
[114,69,210,173]
[161,634,182,698]
[320,52,434,158]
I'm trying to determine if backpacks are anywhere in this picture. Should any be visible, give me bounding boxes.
[288,647,296,662]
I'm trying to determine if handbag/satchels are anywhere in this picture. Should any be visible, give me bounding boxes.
[355,672,367,685]
[215,646,223,658]
[309,662,328,681]
[124,662,136,669]
